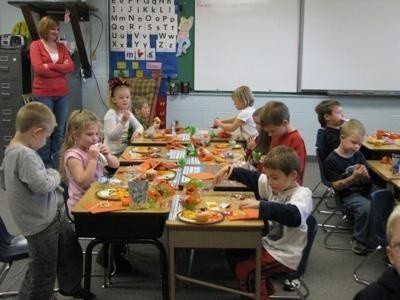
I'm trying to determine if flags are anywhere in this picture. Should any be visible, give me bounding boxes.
[63,8,71,23]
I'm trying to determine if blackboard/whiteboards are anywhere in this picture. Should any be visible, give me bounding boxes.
[107,0,400,97]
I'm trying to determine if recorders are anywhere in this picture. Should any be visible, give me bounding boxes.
[0,34,28,50]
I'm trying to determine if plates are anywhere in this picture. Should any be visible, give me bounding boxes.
[158,169,178,179]
[177,208,225,226]
[221,153,244,161]
[215,142,244,150]
[132,146,161,154]
[164,127,184,134]
[95,188,127,201]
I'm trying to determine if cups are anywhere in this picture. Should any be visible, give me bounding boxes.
[69,41,76,51]
[180,81,190,93]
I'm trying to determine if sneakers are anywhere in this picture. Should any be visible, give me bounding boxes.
[350,237,367,255]
[59,287,95,300]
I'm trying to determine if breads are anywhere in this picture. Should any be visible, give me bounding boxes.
[154,116,161,124]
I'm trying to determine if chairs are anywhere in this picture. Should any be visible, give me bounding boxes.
[353,189,395,286]
[0,215,60,296]
[250,215,318,300]
[308,147,376,252]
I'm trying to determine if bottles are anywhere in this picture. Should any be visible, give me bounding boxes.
[391,153,400,177]
[169,76,180,96]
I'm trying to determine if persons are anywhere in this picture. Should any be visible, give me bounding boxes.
[351,197,400,299]
[127,95,163,145]
[321,118,381,255]
[315,100,351,163]
[4,101,97,300]
[101,80,144,173]
[211,85,307,186]
[215,144,313,300]
[59,106,146,278]
[29,15,75,170]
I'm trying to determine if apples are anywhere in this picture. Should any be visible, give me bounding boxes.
[147,190,160,201]
[190,179,202,188]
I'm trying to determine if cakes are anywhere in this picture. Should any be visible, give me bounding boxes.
[146,169,156,179]
[194,207,209,222]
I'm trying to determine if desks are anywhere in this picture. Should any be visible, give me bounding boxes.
[361,131,400,188]
[71,125,264,296]
